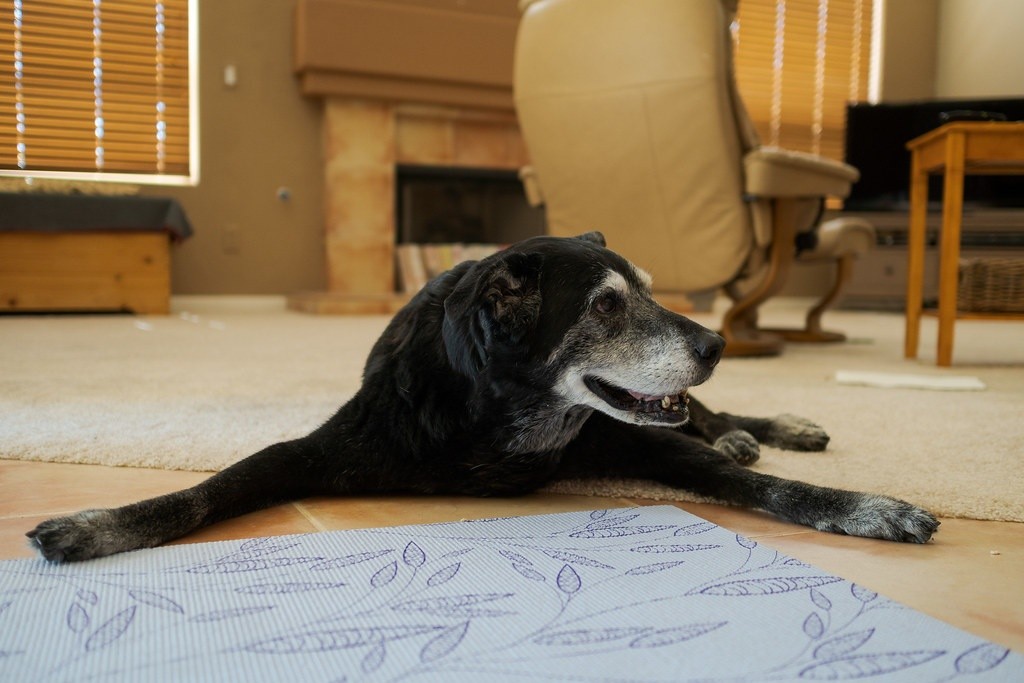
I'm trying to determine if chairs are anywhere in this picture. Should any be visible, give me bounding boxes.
[511,0,877,360]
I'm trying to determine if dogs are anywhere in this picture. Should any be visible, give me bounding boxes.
[23,230,942,566]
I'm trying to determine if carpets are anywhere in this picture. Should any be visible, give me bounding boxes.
[0,501,1024,683]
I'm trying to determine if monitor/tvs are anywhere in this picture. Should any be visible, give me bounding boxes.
[840,97,1024,248]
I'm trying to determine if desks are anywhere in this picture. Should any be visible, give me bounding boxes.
[903,122,1024,366]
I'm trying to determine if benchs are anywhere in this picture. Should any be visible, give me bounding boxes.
[0,191,195,314]
